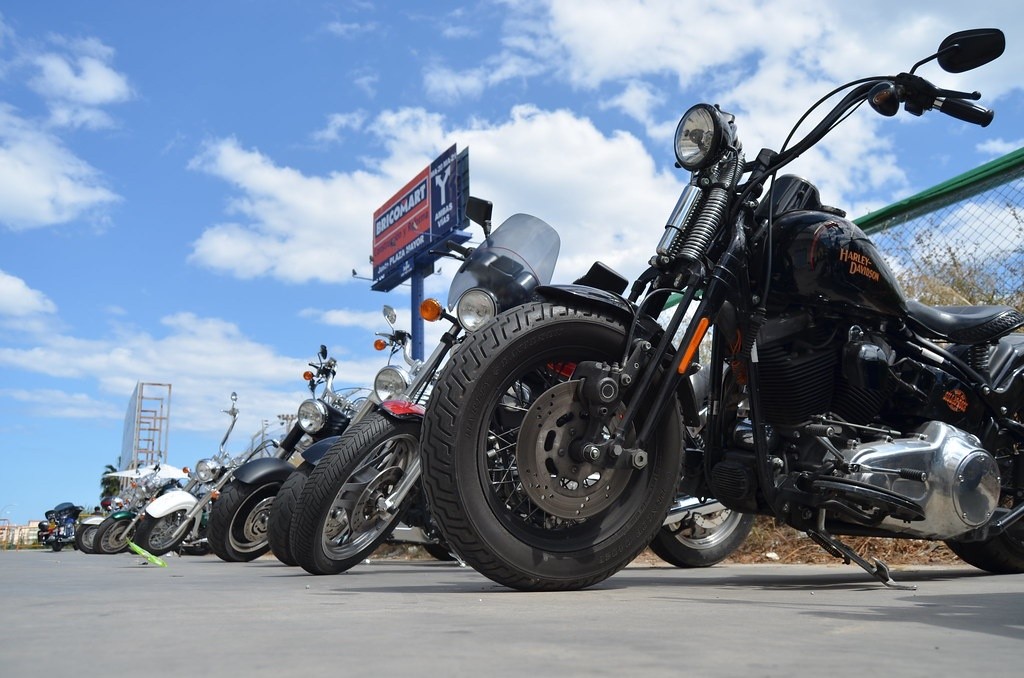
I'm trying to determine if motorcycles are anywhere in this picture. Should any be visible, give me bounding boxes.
[267,304,440,566]
[419,28,1024,591]
[289,196,760,575]
[37,344,374,564]
